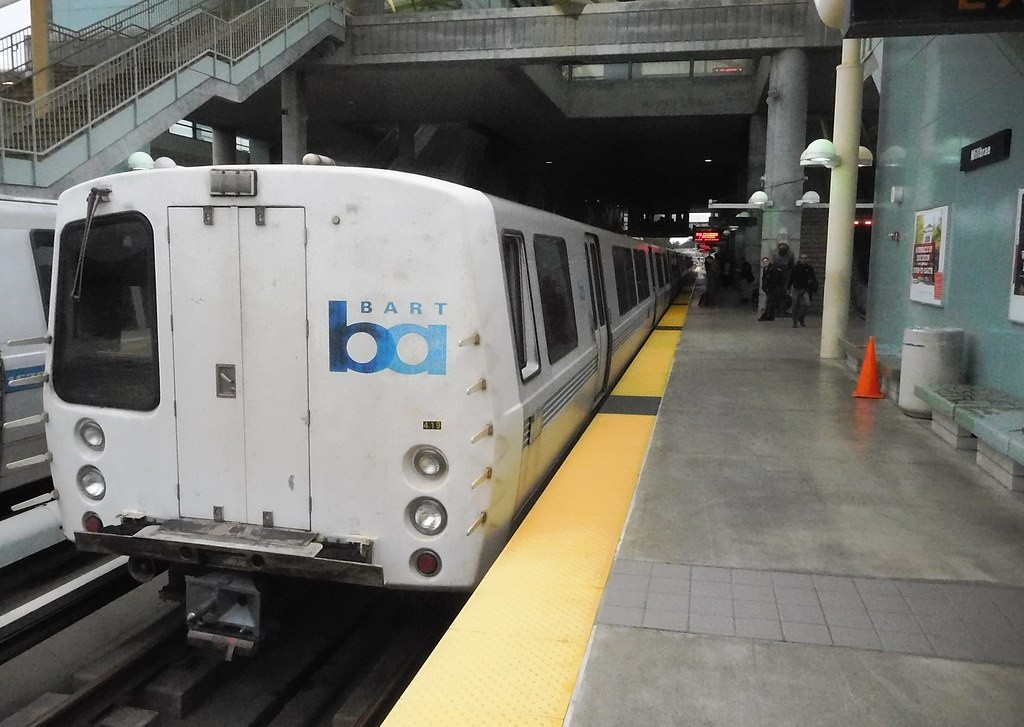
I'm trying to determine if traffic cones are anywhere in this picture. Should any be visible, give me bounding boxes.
[851,336,884,400]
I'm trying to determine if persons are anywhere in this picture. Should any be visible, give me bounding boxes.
[758,244,817,329]
[698,252,755,306]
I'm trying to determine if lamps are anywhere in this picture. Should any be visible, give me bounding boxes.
[858,146,873,168]
[800,140,841,170]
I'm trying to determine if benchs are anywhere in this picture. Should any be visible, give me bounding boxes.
[914,383,1024,493]
[837,335,903,403]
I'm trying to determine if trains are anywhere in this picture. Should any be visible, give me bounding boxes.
[1,194,58,489]
[39,151,697,664]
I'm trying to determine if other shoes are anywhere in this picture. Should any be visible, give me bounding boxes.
[793,320,797,328]
[800,320,806,327]
[766,314,775,321]
[758,314,767,321]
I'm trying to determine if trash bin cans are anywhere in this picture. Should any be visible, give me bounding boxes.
[898,326,971,420]
